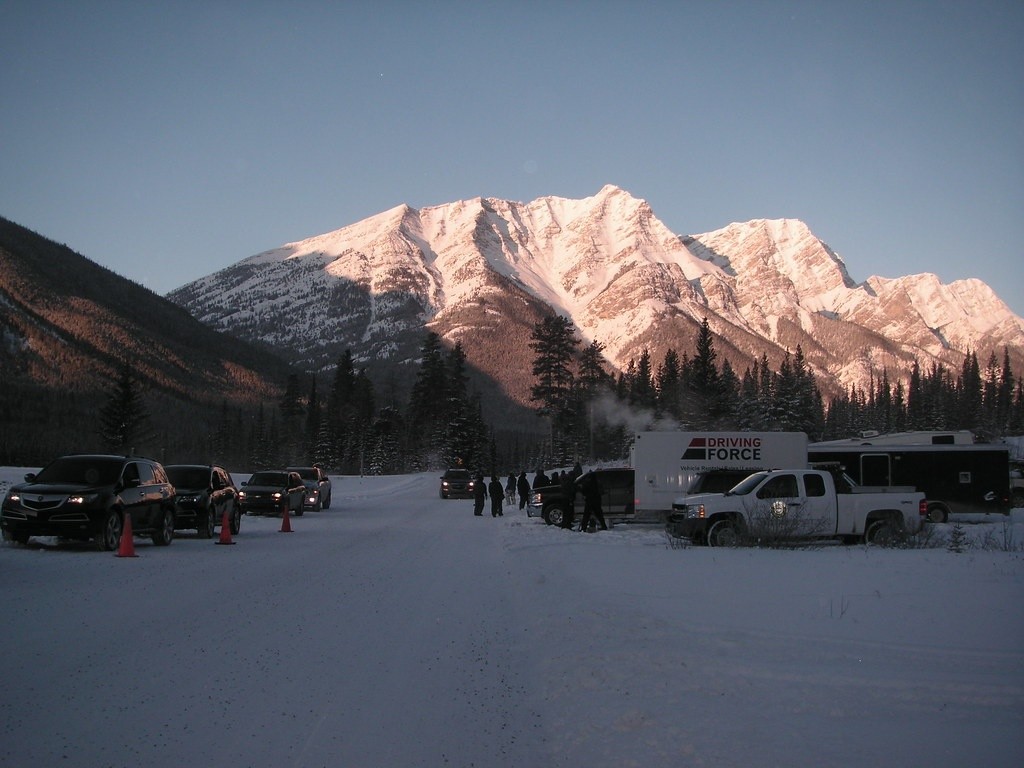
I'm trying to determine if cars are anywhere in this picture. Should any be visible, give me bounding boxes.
[238,471,307,516]
[687,468,763,493]
[287,467,331,512]
[526,467,635,527]
[439,469,475,498]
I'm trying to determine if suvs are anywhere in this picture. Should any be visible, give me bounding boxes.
[0,454,178,551]
[150,464,242,539]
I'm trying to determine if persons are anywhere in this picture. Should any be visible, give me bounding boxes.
[518,471,530,510]
[505,472,516,505]
[489,474,505,517]
[533,462,608,532]
[473,474,487,516]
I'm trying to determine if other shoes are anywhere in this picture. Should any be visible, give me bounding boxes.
[598,527,607,530]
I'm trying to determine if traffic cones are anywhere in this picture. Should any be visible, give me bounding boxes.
[278,505,295,532]
[114,513,140,557]
[215,512,236,544]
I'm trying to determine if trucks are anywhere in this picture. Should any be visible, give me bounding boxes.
[807,443,1010,524]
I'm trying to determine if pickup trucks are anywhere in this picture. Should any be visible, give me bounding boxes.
[665,470,927,548]
